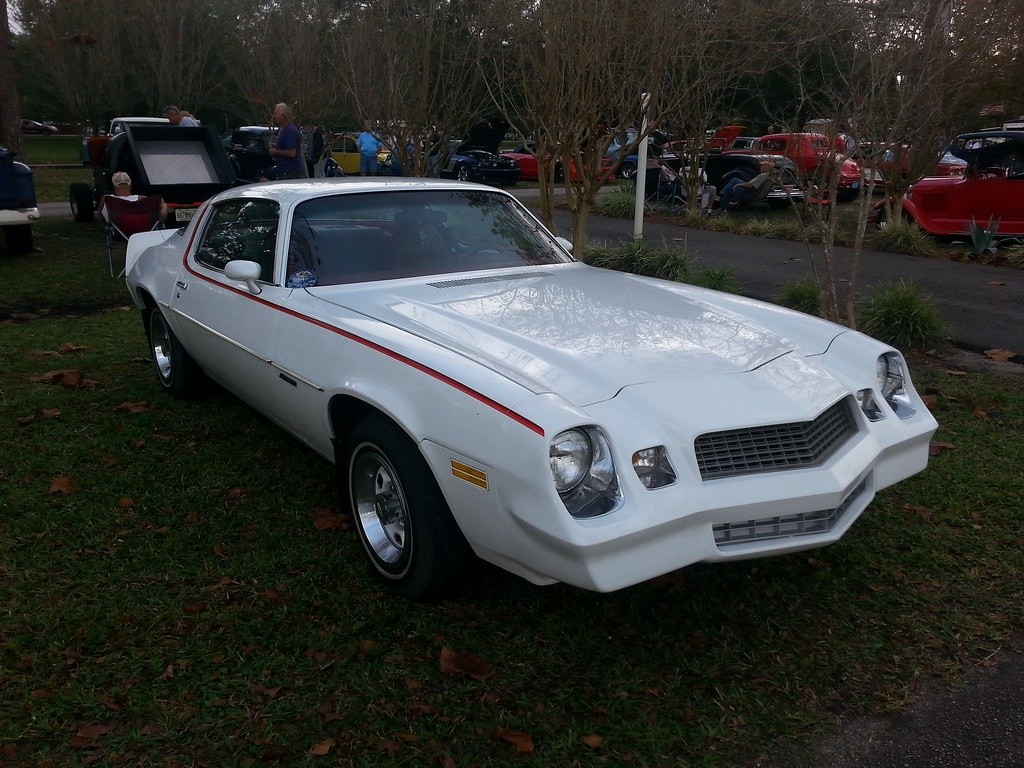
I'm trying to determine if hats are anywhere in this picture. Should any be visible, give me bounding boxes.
[112,172,131,187]
[759,161,772,165]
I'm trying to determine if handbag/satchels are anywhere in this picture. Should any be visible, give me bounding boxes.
[314,147,328,178]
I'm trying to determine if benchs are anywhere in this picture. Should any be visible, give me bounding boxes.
[313,223,387,276]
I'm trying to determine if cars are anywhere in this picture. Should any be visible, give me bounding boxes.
[875,125,1024,238]
[67,122,969,250]
[1,146,40,258]
[21,119,59,137]
[125,177,938,604]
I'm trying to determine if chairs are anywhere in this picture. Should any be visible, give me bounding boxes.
[630,161,711,215]
[719,170,780,217]
[389,213,452,263]
[800,177,832,224]
[101,194,161,279]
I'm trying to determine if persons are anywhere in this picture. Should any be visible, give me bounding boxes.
[102,172,167,223]
[269,102,302,179]
[426,126,442,178]
[323,129,333,144]
[356,120,383,175]
[718,161,773,213]
[164,105,201,127]
[768,126,774,134]
[302,119,323,179]
[647,120,716,217]
